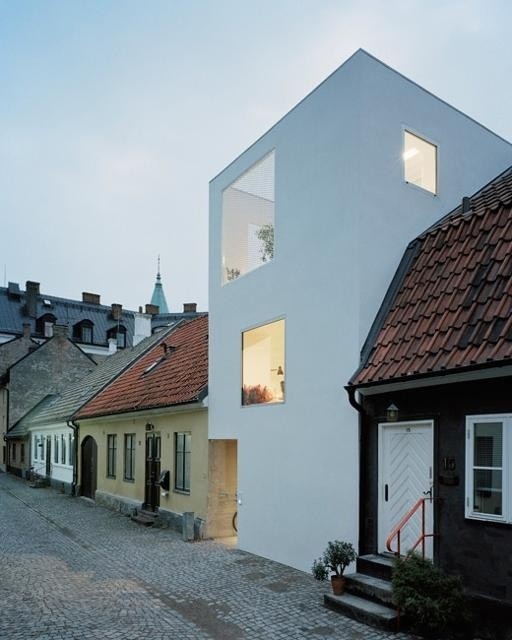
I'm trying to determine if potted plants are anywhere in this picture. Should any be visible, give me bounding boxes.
[311,539,359,596]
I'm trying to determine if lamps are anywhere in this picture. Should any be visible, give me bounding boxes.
[270,365,283,375]
[387,404,399,421]
